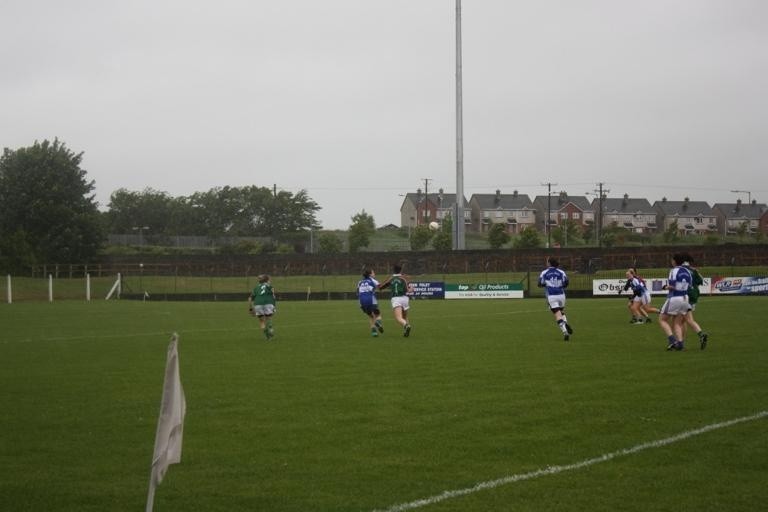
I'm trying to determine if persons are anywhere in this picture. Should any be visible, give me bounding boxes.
[618,266,654,324]
[356,267,393,337]
[657,253,694,352]
[380,265,414,337]
[679,254,709,350]
[537,256,573,341]
[247,273,277,343]
[625,270,662,325]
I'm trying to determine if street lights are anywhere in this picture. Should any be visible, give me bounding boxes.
[132,226,150,256]
[584,192,599,249]
[549,190,570,248]
[731,190,753,240]
[398,192,414,252]
[437,194,444,233]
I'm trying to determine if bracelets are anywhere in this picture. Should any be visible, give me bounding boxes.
[249,308,253,311]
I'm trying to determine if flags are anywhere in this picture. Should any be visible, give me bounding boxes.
[150,333,187,486]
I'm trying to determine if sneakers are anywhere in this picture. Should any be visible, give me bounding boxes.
[564,324,572,341]
[629,316,652,324]
[700,334,708,350]
[267,324,275,342]
[666,340,688,352]
[403,325,411,337]
[371,321,385,337]
